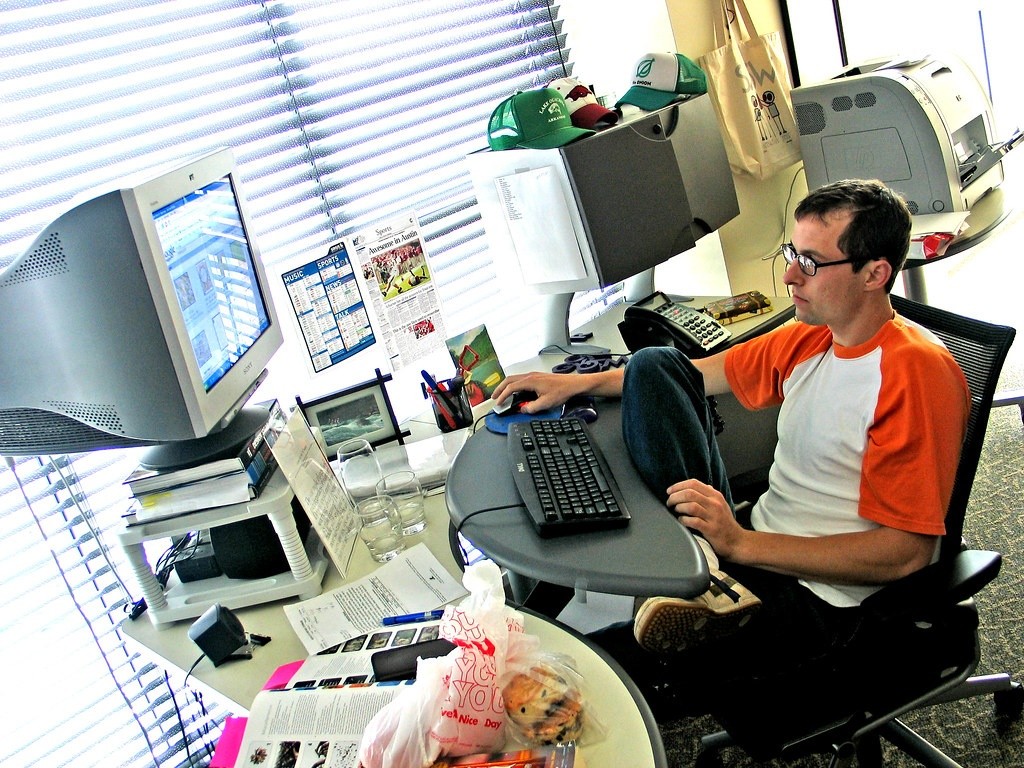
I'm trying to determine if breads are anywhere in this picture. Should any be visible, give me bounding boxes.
[501,666,586,745]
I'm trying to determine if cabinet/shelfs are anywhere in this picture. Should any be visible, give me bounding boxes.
[466,96,741,293]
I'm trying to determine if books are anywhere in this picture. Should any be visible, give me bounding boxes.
[704,290,773,325]
[121,399,288,527]
[234,621,439,768]
[431,740,587,768]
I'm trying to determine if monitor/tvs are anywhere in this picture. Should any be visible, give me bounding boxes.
[0,146,283,473]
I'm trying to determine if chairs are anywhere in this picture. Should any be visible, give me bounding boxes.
[678,292,1024,768]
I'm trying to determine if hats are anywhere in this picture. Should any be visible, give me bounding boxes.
[486,88,596,150]
[613,51,708,110]
[544,77,619,129]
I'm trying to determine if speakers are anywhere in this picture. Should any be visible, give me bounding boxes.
[188,603,253,668]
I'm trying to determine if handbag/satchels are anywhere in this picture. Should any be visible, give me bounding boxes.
[350,558,587,768]
[699,0,804,183]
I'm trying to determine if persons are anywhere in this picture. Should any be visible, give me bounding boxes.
[414,319,434,338]
[491,181,971,728]
[364,245,428,296]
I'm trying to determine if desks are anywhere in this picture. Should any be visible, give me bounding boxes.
[898,184,1024,417]
[123,294,798,768]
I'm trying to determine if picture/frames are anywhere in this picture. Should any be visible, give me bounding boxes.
[289,368,411,463]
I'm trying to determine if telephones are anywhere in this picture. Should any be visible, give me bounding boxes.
[617,289,732,359]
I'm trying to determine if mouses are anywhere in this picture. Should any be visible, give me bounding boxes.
[566,408,598,425]
[492,390,538,418]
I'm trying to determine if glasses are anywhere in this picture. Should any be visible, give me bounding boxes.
[780,243,870,277]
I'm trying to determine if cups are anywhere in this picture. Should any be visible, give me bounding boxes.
[336,439,389,528]
[427,379,473,433]
[353,495,406,563]
[376,470,427,536]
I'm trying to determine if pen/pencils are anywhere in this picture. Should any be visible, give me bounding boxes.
[417,368,473,432]
[382,609,451,624]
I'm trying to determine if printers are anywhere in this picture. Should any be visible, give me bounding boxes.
[790,48,1024,215]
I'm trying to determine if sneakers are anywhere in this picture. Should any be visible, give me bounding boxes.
[634,566,763,659]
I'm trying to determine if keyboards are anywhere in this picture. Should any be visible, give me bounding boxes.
[508,417,631,538]
